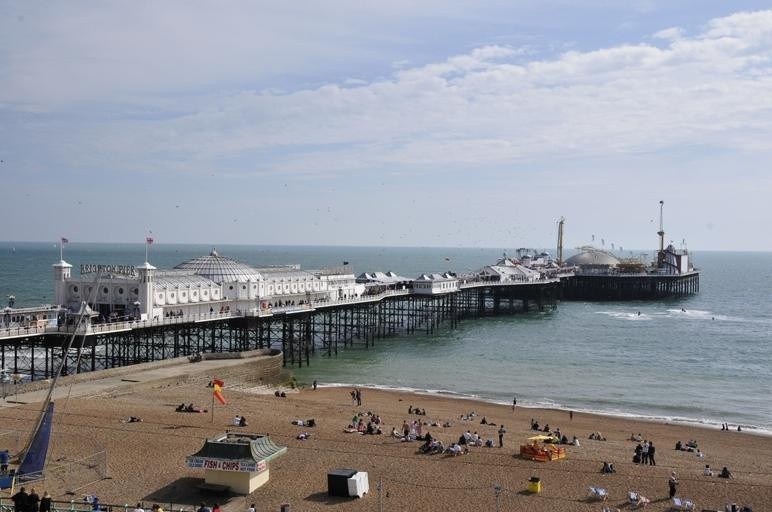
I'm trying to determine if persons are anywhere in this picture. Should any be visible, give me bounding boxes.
[534,410,605,447]
[601,434,756,512]
[293,418,316,427]
[235,415,248,426]
[13,488,257,512]
[350,389,362,406]
[291,379,297,388]
[208,381,212,387]
[275,391,285,397]
[175,403,208,412]
[353,405,506,457]
[299,434,309,440]
[313,381,318,388]
[2,260,576,329]
[721,423,742,431]
[512,396,518,408]
[1,450,10,471]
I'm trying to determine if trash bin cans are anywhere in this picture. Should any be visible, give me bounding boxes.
[527,477,541,493]
